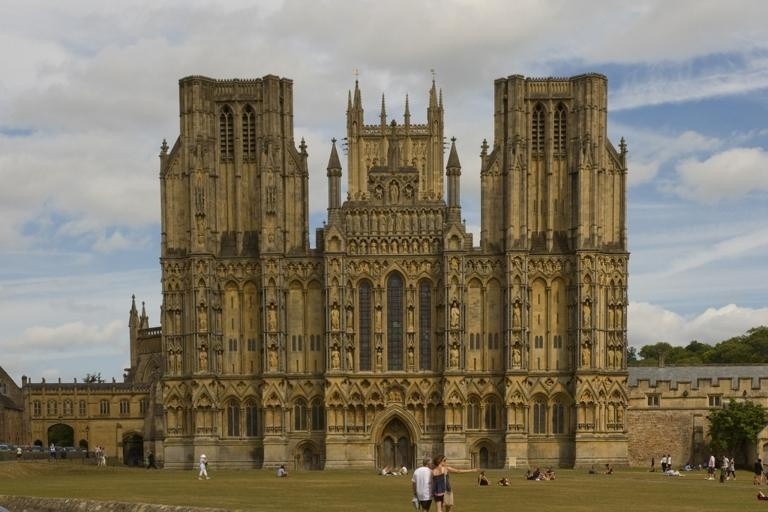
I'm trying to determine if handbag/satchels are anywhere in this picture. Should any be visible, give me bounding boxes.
[442,492,454,507]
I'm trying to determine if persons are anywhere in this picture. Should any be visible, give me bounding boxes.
[382,463,408,476]
[650,454,684,477]
[412,454,480,512]
[479,472,489,486]
[95,444,106,468]
[757,490,768,501]
[707,454,736,482]
[753,459,764,486]
[278,464,288,477]
[606,464,613,475]
[684,463,692,472]
[525,468,556,481]
[49,442,58,462]
[497,477,510,486]
[198,454,211,481]
[16,447,23,460]
[147,451,157,469]
